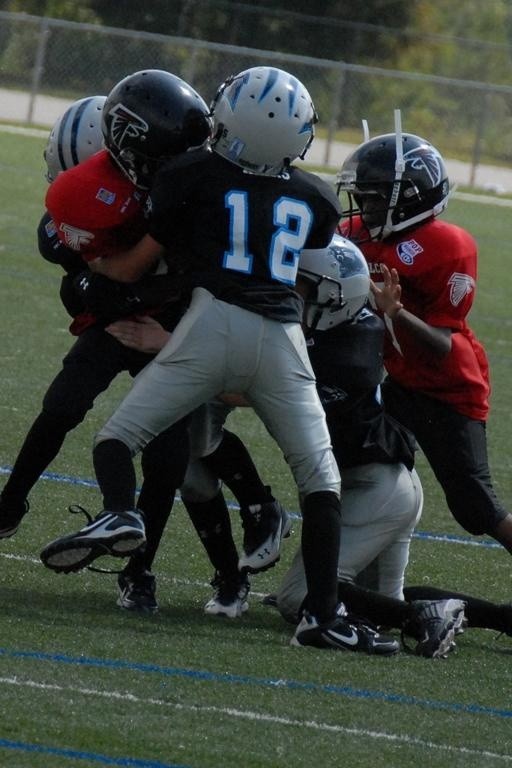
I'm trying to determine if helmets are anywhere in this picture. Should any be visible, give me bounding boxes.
[290,228,373,344]
[48,89,106,186]
[327,132,450,246]
[205,62,319,179]
[98,68,215,192]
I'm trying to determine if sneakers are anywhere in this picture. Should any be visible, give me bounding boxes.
[112,559,161,619]
[2,488,31,537]
[286,605,402,661]
[202,579,255,618]
[407,592,468,658]
[236,490,294,576]
[36,506,149,577]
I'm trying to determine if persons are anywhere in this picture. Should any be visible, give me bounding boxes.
[0,66,512,657]
